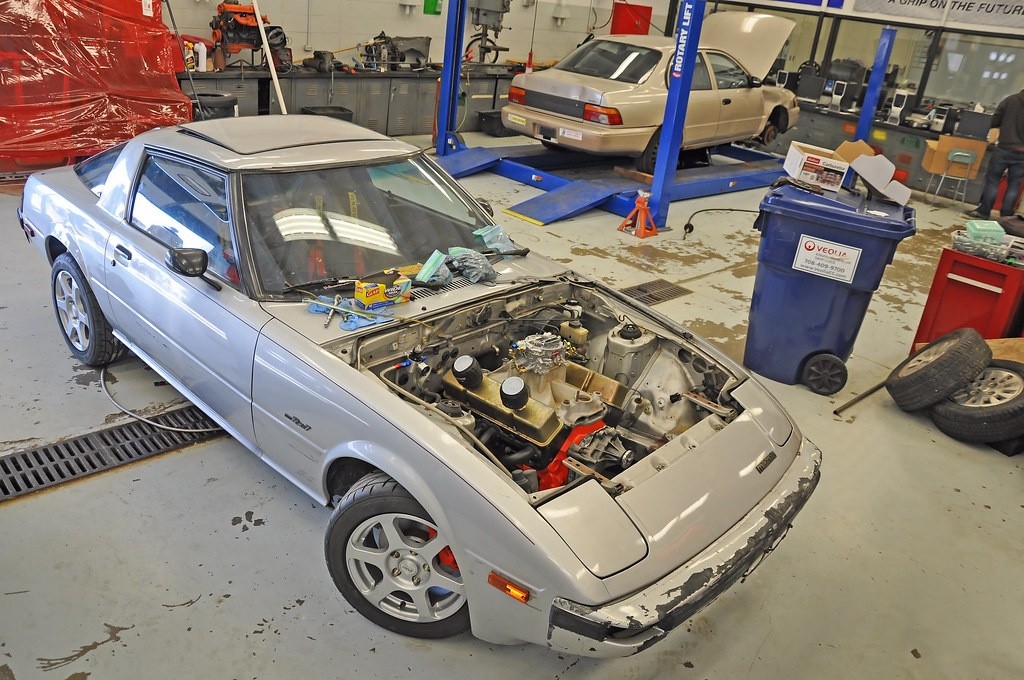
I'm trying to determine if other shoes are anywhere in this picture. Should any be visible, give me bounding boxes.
[966,209,990,220]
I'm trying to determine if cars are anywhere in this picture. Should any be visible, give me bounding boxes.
[502,10,802,157]
[17,114,822,663]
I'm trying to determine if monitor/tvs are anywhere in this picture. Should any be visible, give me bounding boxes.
[956,109,992,138]
[796,74,827,101]
[855,85,888,112]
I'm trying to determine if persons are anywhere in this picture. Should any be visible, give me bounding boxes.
[959,90,1024,221]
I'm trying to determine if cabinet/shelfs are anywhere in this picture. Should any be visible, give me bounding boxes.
[175,70,835,139]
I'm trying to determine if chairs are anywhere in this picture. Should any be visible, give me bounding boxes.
[924,148,977,211]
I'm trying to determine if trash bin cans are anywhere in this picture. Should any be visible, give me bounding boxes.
[742,175,919,398]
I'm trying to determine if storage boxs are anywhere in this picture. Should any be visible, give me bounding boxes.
[951,230,1017,262]
[921,135,988,181]
[965,219,1006,245]
[782,139,912,206]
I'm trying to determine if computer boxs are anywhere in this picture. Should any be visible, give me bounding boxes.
[930,106,958,134]
[888,89,917,125]
[829,80,857,112]
[775,69,800,94]
